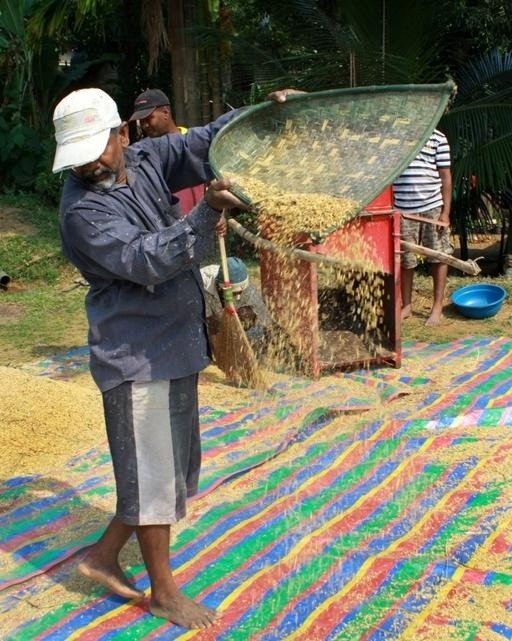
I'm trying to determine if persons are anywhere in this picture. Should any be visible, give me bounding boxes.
[49,81,309,630]
[126,85,227,261]
[198,255,274,392]
[390,125,456,329]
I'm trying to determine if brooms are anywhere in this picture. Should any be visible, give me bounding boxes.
[208,236,261,390]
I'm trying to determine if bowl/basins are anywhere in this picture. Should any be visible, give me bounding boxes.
[449,284,506,320]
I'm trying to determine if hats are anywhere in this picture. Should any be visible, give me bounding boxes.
[127,88,172,124]
[50,86,124,174]
[216,256,251,294]
